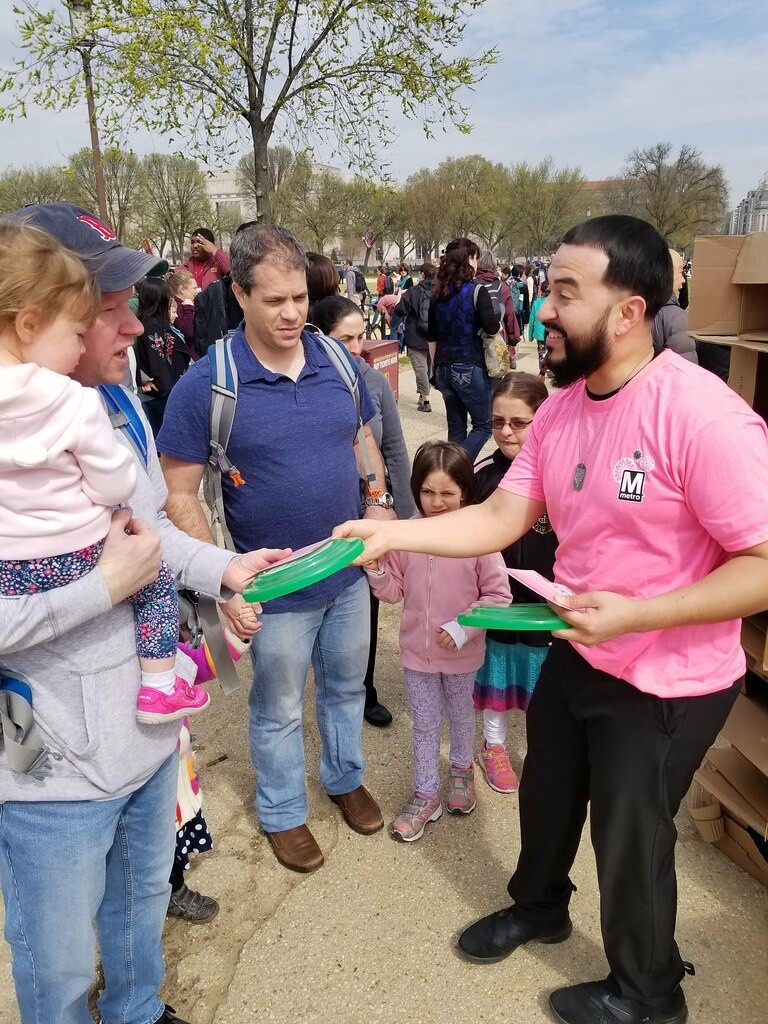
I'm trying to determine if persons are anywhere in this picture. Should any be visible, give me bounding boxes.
[304,295,417,726]
[427,238,503,467]
[302,251,337,332]
[474,371,560,794]
[0,203,293,1024]
[339,258,441,413]
[159,221,400,871]
[471,249,555,383]
[333,215,768,1024]
[361,440,511,840]
[648,247,700,368]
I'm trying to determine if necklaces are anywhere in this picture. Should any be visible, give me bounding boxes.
[571,347,654,491]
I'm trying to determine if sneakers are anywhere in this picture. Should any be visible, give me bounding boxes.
[457,905,573,965]
[169,882,219,926]
[548,978,688,1024]
[418,396,423,404]
[389,789,444,842]
[444,759,477,816]
[478,737,520,794]
[417,404,432,411]
[135,675,210,725]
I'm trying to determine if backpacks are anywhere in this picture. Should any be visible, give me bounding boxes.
[345,270,366,292]
[418,283,438,338]
[474,278,504,321]
[511,281,524,316]
[384,276,394,295]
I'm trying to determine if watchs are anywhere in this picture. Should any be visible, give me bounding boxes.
[365,492,395,511]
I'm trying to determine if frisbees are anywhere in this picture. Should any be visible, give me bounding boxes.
[239,535,365,604]
[456,602,575,632]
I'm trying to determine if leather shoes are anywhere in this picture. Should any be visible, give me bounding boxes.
[327,784,385,836]
[364,703,393,727]
[265,824,325,874]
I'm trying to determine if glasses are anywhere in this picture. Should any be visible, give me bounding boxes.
[484,413,534,431]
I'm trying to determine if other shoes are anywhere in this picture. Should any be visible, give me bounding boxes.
[546,368,555,378]
[158,1005,192,1024]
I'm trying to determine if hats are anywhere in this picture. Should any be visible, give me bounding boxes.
[533,260,542,266]
[0,201,169,293]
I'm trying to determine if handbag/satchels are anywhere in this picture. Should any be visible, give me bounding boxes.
[474,283,511,379]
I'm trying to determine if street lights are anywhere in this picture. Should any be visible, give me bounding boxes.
[68,0,110,228]
